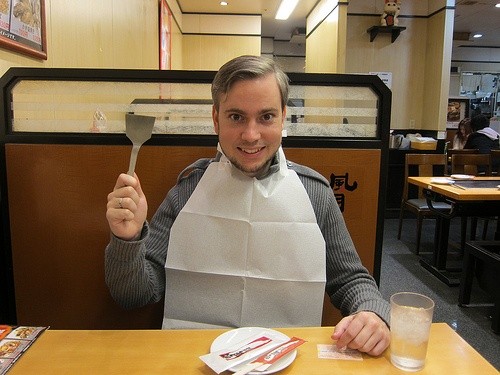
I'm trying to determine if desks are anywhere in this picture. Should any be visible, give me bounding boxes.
[4,321,500,375]
[406,174,500,288]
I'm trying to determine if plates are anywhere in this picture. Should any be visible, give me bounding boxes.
[210,327,297,375]
[429,177,455,184]
[451,174,476,179]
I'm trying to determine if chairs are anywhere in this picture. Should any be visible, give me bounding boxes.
[397,149,500,335]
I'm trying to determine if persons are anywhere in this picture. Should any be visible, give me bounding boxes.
[453,118,473,149]
[105,54,392,356]
[462,114,500,172]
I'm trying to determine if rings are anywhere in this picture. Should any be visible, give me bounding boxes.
[120,198,123,208]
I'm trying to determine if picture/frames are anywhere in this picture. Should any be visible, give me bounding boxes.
[0,0,47,61]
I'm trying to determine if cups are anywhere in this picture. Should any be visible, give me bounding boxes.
[390,291,435,372]
[390,135,404,149]
